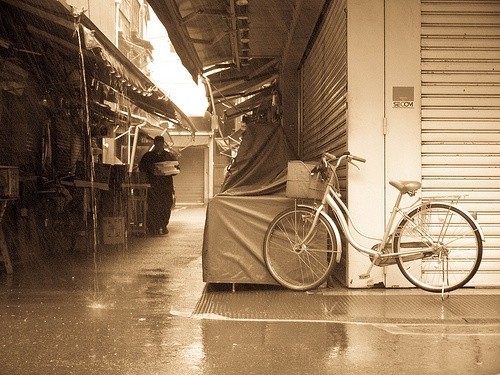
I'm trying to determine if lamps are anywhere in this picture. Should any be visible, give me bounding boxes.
[235,0,251,67]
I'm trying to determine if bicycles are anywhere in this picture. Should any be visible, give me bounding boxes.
[263,150,486,303]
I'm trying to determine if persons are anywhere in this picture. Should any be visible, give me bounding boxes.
[141,136,181,235]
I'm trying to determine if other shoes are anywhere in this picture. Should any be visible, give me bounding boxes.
[163,227,169,234]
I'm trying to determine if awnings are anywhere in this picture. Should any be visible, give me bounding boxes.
[224,90,273,120]
[146,0,232,85]
[0,0,198,140]
[203,62,271,102]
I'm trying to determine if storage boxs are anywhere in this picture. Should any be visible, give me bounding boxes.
[75,160,112,183]
[103,217,125,245]
[0,166,19,197]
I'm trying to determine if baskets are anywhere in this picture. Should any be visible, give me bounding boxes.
[285,159,333,201]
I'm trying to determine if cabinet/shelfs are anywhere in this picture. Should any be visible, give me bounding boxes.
[121,184,151,238]
